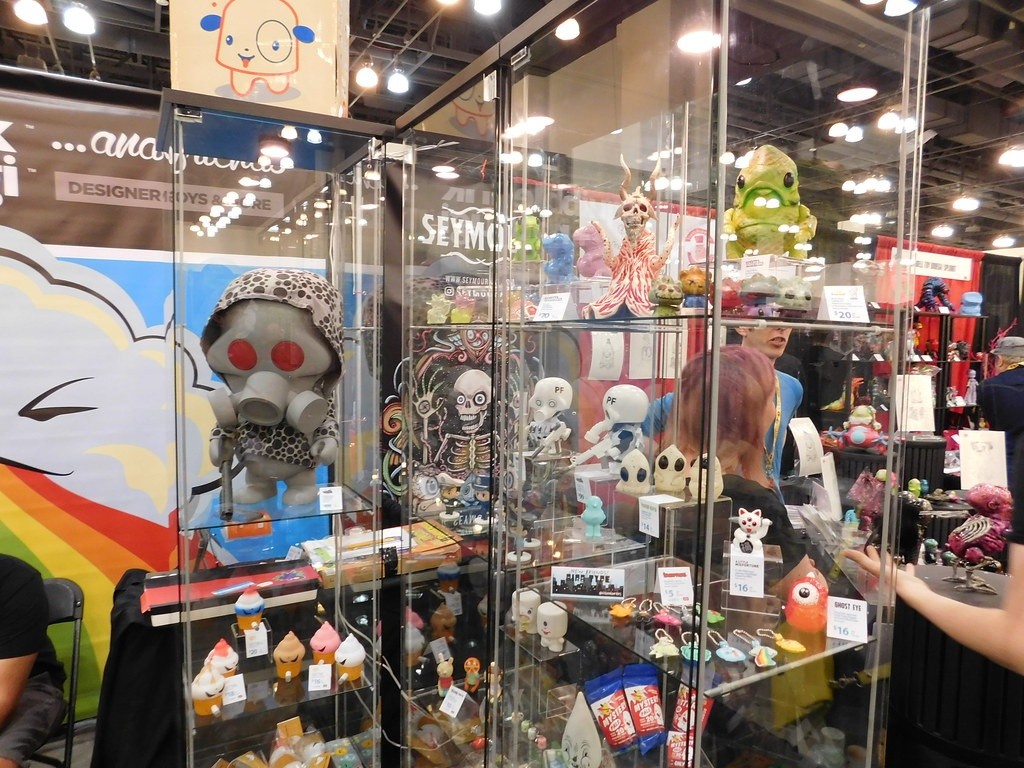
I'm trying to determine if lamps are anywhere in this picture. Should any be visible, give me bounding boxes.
[884,0,920,17]
[356,61,377,87]
[61,0,96,35]
[387,66,409,93]
[835,81,878,102]
[677,16,714,53]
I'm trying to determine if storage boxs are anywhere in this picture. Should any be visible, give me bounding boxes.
[143,560,318,627]
[319,521,463,589]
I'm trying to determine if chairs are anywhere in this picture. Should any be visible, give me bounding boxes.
[24,577,85,768]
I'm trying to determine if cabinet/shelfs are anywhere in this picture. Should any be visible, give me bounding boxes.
[151,85,400,768]
[394,0,931,768]
[836,306,990,430]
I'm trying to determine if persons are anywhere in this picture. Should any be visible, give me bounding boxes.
[528,377,572,455]
[845,434,1024,678]
[200,268,343,505]
[977,337,1024,491]
[638,326,802,506]
[802,330,844,406]
[964,369,979,405]
[585,384,646,474]
[0,554,65,768]
[640,345,795,583]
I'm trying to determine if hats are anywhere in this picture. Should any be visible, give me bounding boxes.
[989,336,1023,357]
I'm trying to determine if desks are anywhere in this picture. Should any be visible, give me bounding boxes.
[860,565,1024,768]
[925,490,1007,573]
[829,444,896,479]
[886,432,951,494]
[89,555,545,768]
